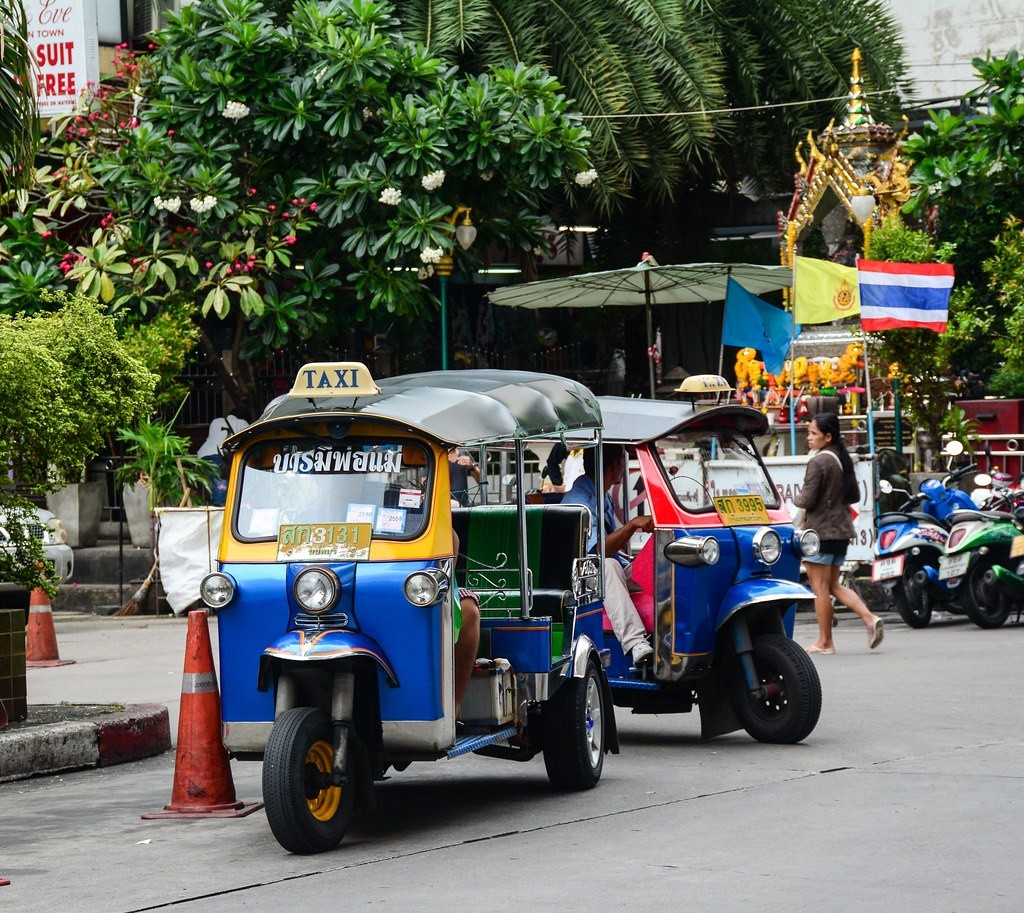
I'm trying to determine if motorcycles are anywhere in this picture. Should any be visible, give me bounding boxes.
[446,373,823,746]
[201,358,622,855]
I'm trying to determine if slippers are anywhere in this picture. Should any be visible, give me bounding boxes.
[866,616,884,649]
[804,644,836,655]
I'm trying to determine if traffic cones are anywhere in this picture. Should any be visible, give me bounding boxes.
[24,558,76,668]
[139,610,264,819]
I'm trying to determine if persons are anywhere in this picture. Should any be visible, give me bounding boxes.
[421,447,480,507]
[311,445,481,720]
[542,443,654,669]
[795,412,884,654]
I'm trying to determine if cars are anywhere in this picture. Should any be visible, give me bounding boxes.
[0,500,74,586]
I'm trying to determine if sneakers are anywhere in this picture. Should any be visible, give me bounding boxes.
[630,638,654,666]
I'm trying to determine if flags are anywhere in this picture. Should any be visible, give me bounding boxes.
[795,254,860,324]
[722,276,801,375]
[855,258,955,333]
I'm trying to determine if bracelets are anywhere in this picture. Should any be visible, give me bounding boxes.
[469,465,476,471]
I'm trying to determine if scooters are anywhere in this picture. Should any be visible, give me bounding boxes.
[937,436,1024,631]
[871,440,983,630]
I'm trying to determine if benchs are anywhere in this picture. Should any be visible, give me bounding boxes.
[449,503,593,656]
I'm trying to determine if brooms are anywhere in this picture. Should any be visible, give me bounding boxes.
[111,488,191,616]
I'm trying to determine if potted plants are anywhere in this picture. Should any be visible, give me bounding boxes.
[40,298,220,548]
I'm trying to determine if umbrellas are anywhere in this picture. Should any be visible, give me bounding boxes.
[490,256,791,399]
